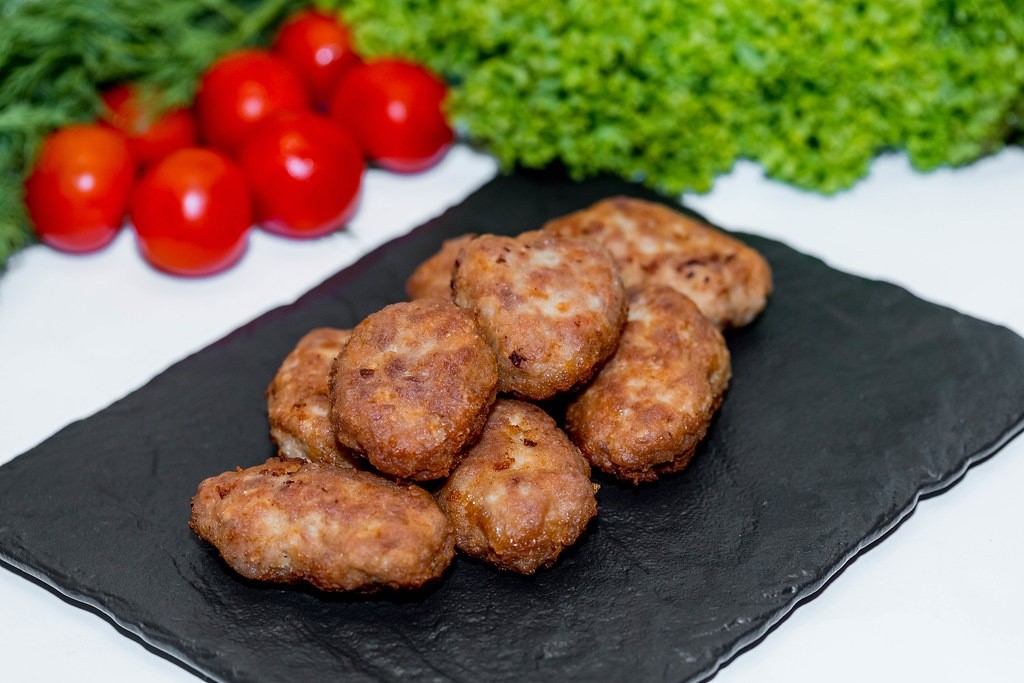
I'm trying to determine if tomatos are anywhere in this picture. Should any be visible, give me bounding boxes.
[24,13,455,274]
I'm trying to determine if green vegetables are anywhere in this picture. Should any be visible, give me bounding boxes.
[315,0,1024,198]
[0,0,304,296]
